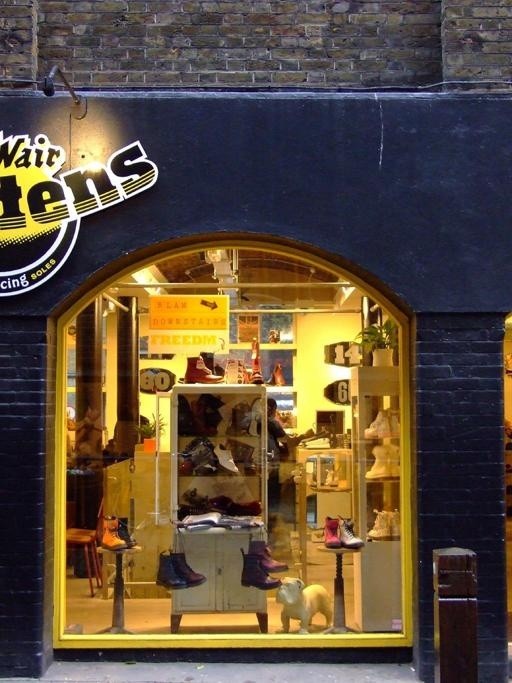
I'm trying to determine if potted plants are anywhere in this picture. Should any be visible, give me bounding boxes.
[132,413,167,454]
[356,318,398,366]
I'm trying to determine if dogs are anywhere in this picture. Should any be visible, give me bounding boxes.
[275,576,333,635]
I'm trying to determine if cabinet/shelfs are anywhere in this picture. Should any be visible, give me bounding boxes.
[351,365,406,631]
[170,383,268,633]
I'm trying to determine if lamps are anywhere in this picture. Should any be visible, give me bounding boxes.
[41,61,88,120]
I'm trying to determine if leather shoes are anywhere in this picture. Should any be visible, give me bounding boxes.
[213,443,241,475]
[273,365,287,385]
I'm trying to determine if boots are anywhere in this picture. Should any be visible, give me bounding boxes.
[322,514,364,549]
[365,445,400,481]
[185,357,223,383]
[367,510,400,541]
[230,407,241,436]
[240,535,289,589]
[102,516,137,550]
[157,551,206,589]
[251,338,264,384]
[364,409,400,438]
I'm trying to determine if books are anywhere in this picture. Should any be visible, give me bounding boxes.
[168,511,265,531]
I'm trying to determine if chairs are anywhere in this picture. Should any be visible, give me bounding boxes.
[66,487,109,598]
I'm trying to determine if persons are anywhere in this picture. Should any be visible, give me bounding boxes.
[267,397,315,538]
